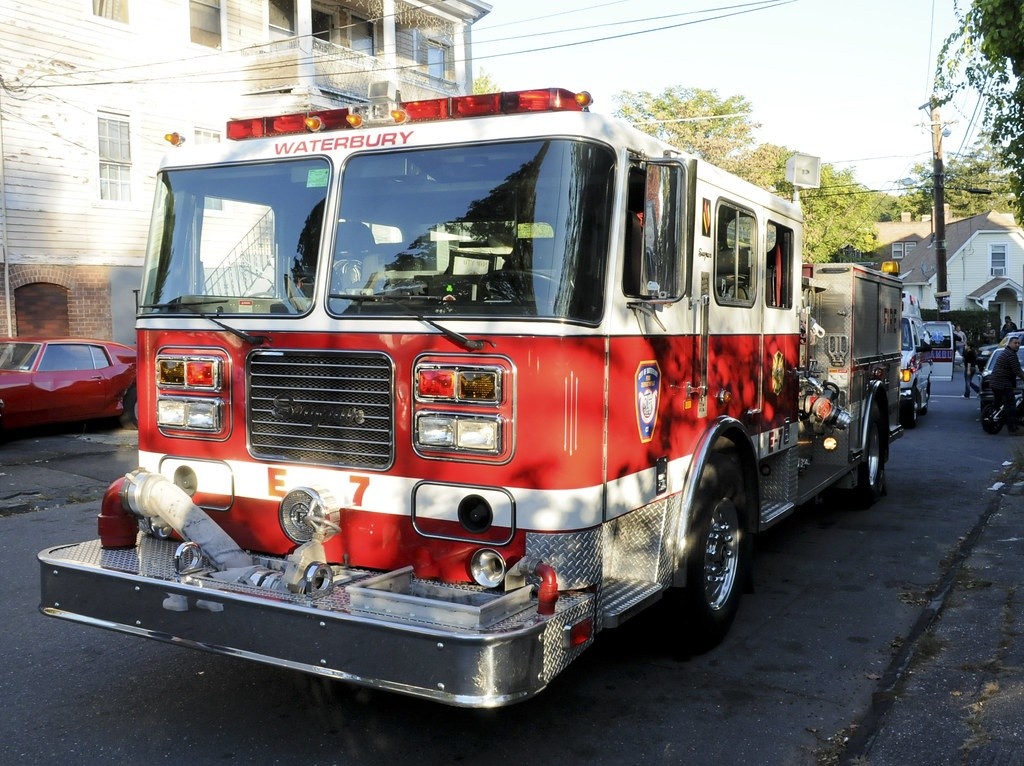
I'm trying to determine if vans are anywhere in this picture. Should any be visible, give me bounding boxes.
[901,317,956,429]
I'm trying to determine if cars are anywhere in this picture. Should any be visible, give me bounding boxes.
[0,336,138,431]
[973,330,1024,417]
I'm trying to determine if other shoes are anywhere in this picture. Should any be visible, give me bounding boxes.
[960,395,969,399]
[1008,428,1024,435]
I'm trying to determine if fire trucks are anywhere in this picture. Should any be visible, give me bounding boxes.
[36,84,909,712]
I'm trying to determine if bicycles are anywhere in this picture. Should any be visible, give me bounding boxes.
[979,391,1024,434]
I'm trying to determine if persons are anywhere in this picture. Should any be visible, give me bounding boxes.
[989,337,1024,436]
[982,322,996,345]
[962,341,981,399]
[953,325,967,366]
[1001,316,1017,339]
